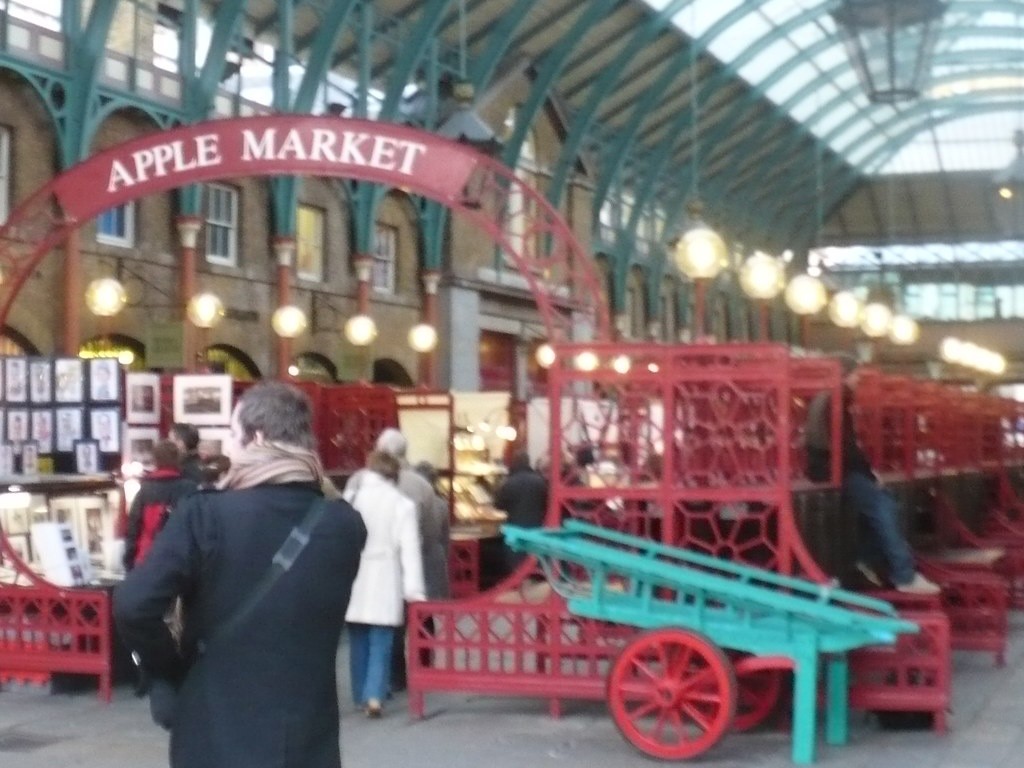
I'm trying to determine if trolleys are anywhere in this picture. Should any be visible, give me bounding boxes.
[496,517,924,766]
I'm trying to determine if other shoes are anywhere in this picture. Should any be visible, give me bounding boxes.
[366,697,381,720]
[896,572,940,595]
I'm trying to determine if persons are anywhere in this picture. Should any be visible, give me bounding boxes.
[378,427,449,691]
[122,442,192,576]
[417,460,450,556]
[114,382,369,768]
[340,451,428,717]
[492,450,549,567]
[573,448,595,510]
[168,422,231,490]
[794,354,914,593]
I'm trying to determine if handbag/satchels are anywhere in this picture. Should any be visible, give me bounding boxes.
[147,674,180,730]
